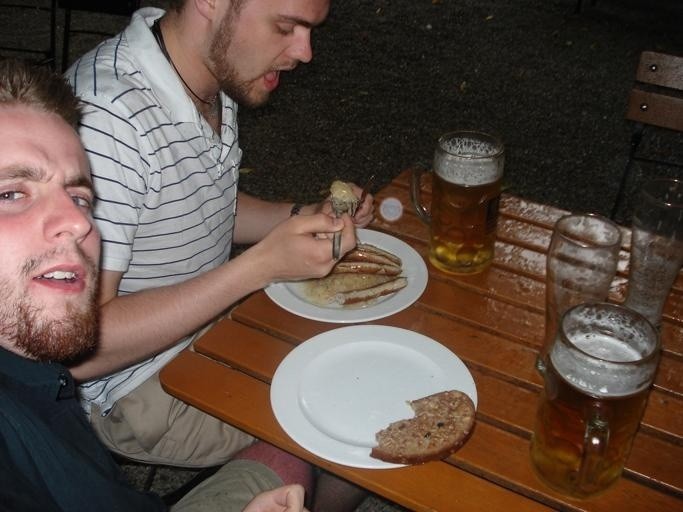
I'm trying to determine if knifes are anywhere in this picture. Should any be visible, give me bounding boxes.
[353,172,376,220]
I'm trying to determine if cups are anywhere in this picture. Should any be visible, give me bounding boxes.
[412,131,507,276]
[537,212,622,377]
[626,178,683,327]
[531,302,662,500]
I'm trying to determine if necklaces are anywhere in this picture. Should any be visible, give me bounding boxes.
[151,20,221,118]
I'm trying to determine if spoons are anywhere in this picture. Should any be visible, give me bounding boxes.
[330,188,350,261]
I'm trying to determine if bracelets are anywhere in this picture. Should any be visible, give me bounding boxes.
[291,201,305,216]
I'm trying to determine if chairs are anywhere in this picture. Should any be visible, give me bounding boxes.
[610,46,683,226]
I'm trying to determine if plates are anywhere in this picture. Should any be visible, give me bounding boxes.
[262,227,428,325]
[269,323,477,471]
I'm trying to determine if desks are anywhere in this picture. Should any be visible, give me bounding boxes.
[161,154,681,510]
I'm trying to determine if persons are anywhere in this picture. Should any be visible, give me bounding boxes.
[0,50,410,512]
[58,1,374,512]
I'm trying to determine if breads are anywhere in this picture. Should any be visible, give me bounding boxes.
[369,390,476,464]
